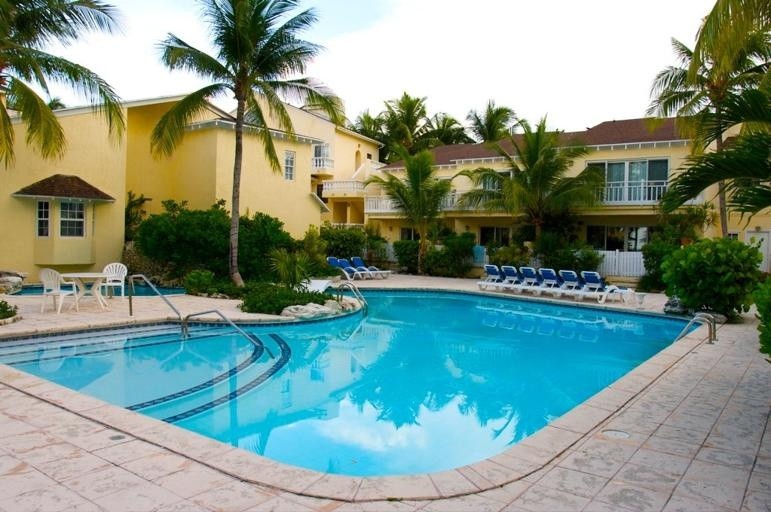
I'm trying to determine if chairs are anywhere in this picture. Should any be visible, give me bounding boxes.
[99,262,127,301]
[327,256,393,281]
[476,264,627,305]
[39,268,78,315]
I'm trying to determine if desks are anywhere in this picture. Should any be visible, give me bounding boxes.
[60,272,108,312]
